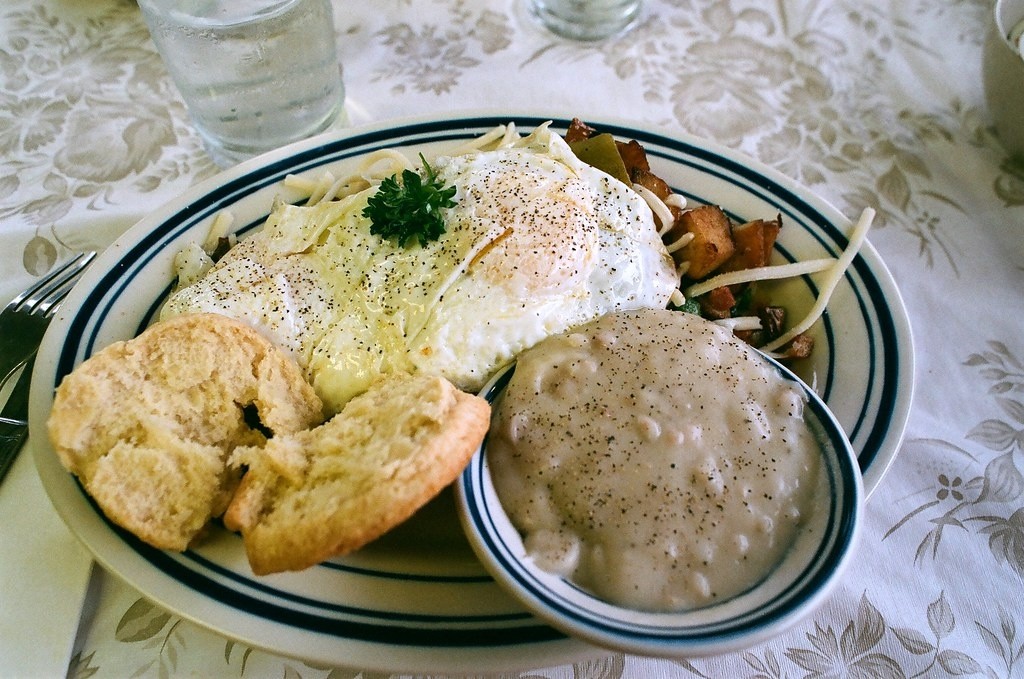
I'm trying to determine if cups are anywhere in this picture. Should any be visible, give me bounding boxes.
[136,2,343,163]
[529,2,640,43]
[982,1,1024,162]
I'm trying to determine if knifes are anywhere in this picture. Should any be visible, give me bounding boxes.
[0,347,39,483]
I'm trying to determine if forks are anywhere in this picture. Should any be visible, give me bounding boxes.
[0,251,98,395]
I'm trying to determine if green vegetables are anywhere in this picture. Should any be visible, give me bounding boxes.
[363,151,459,249]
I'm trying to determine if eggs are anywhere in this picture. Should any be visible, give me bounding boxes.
[154,123,679,411]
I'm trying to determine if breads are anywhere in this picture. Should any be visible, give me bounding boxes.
[46,312,492,576]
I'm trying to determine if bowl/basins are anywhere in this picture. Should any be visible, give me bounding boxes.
[455,342,864,656]
[29,114,916,672]
